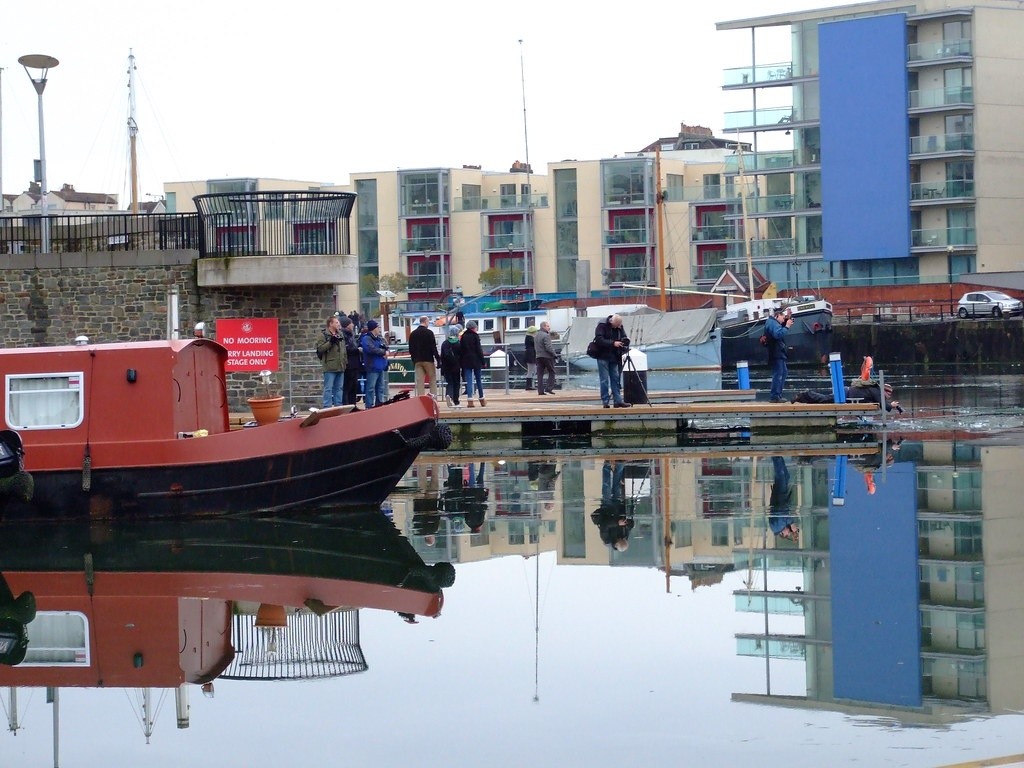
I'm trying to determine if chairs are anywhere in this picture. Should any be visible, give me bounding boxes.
[768,69,785,80]
[934,187,946,198]
[775,200,784,211]
[922,188,929,199]
[777,116,792,124]
[812,237,821,252]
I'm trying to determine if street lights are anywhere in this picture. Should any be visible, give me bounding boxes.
[423,249,432,299]
[664,262,675,312]
[791,257,802,296]
[16,53,62,253]
[507,242,514,285]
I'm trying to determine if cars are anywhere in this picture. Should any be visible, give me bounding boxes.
[957,290,1024,318]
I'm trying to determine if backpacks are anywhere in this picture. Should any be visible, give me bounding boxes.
[851,376,880,389]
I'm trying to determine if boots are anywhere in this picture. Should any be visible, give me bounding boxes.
[467,398,475,408]
[478,396,487,406]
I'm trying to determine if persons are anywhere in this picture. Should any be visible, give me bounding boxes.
[534,322,558,395]
[528,462,539,490]
[456,309,465,325]
[764,311,794,403]
[413,463,443,546]
[538,461,561,510]
[442,463,489,539]
[791,382,899,413]
[317,310,389,412]
[599,461,634,552]
[796,439,901,472]
[408,316,440,403]
[594,314,631,408]
[441,325,476,406]
[768,457,798,539]
[460,320,486,407]
[523,325,539,389]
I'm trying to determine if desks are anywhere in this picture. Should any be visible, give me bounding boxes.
[781,201,791,210]
[927,189,937,200]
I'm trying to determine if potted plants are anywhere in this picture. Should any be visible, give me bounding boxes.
[248,370,284,426]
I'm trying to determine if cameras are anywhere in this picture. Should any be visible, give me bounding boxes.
[619,338,631,347]
[380,344,387,351]
[894,401,906,415]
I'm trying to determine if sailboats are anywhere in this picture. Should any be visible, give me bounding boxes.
[557,131,835,372]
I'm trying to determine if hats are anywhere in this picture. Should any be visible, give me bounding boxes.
[466,320,477,329]
[449,326,460,336]
[367,320,378,332]
[528,326,537,334]
[884,383,893,393]
[338,316,352,328]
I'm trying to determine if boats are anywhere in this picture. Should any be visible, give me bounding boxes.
[363,282,652,369]
[0,504,457,768]
[0,284,438,524]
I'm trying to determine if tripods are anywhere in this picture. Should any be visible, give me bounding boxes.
[605,349,653,410]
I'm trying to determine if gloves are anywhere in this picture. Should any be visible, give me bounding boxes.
[330,336,338,345]
[436,358,442,369]
[484,363,487,369]
[378,344,388,351]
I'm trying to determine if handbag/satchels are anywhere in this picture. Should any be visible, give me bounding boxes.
[590,508,612,534]
[587,335,608,360]
[316,331,329,360]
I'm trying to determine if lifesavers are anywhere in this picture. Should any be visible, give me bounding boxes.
[864,472,876,495]
[862,356,872,381]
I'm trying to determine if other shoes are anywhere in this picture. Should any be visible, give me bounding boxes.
[614,402,631,408]
[791,392,801,404]
[350,407,361,412]
[446,395,453,408]
[797,388,809,397]
[525,387,537,390]
[539,392,547,395]
[454,401,460,406]
[768,398,787,403]
[545,389,555,394]
[461,389,468,396]
[604,404,610,409]
